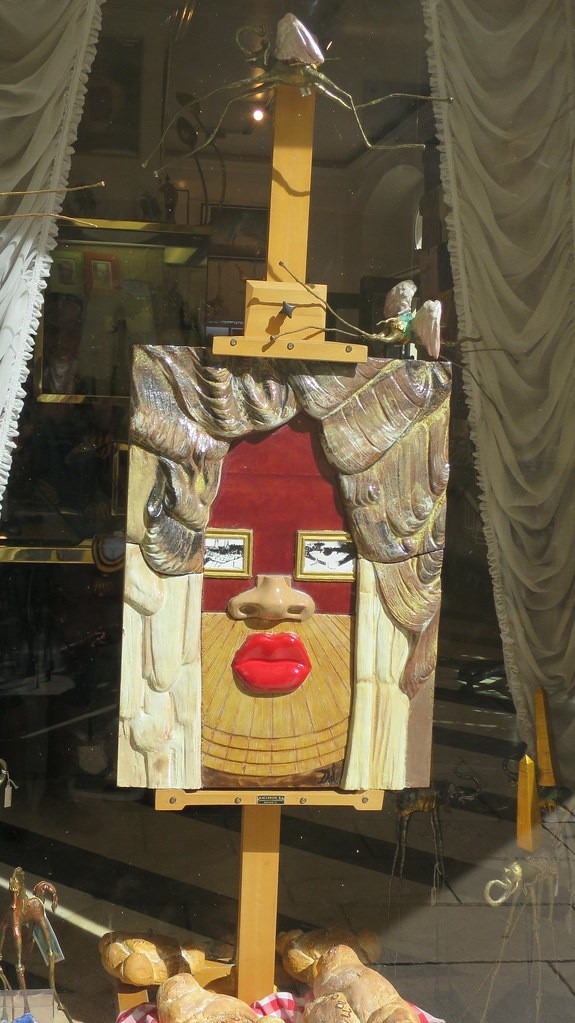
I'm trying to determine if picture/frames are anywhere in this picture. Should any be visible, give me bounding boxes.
[201,203,269,262]
[84,253,119,295]
[49,250,84,293]
